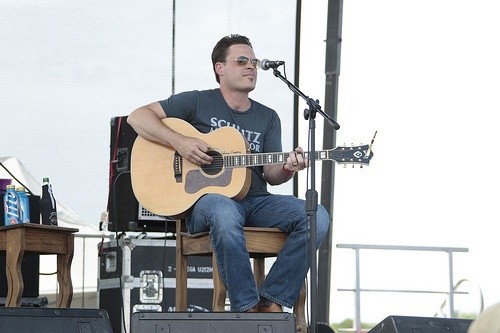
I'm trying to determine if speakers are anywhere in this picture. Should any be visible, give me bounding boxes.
[365,315,474,333]
[108,116,176,232]
[131,311,297,333]
[0,306,114,333]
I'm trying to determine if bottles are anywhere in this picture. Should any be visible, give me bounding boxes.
[3,185,21,226]
[15,187,31,223]
[42,178,57,226]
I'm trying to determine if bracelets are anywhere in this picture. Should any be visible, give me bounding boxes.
[283,163,295,175]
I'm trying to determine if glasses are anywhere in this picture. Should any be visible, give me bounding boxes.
[223,55,262,70]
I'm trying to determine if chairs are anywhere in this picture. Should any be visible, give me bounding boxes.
[175,219,307,333]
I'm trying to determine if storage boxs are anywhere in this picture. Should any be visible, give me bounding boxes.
[96,240,214,333]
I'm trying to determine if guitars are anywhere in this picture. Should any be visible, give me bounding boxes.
[131,117,374,221]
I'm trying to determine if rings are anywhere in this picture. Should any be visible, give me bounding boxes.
[293,162,297,166]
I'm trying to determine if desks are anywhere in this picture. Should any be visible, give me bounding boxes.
[0,223,79,308]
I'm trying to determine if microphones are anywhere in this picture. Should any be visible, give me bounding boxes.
[260,59,285,70]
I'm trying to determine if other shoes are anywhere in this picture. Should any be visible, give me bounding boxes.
[259,301,284,312]
[244,302,260,313]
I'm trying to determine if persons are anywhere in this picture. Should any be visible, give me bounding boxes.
[127,35,331,313]
